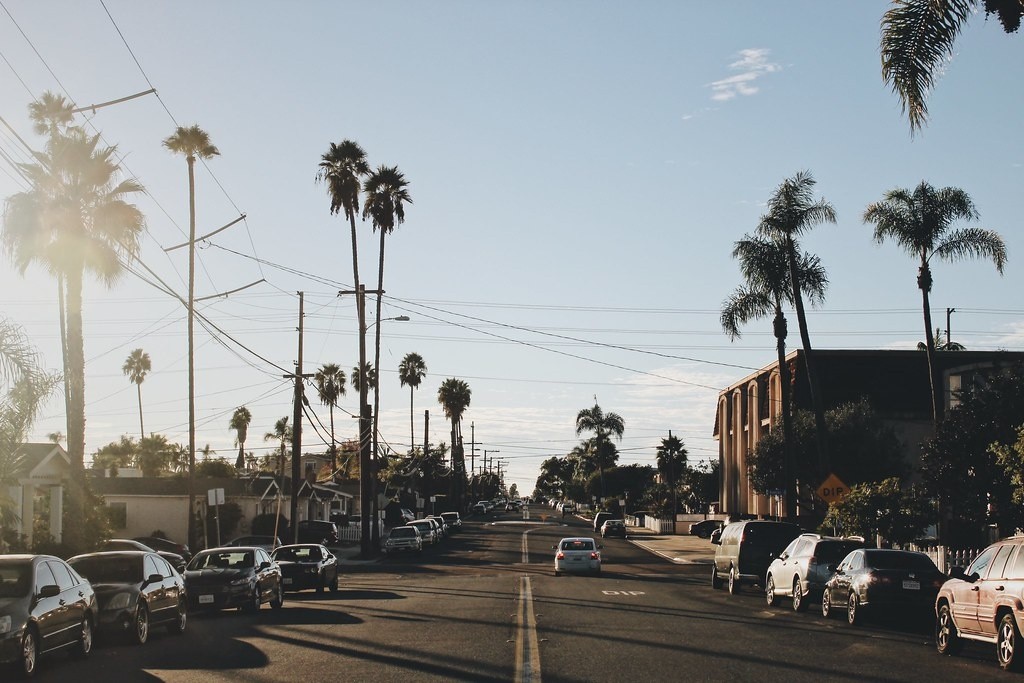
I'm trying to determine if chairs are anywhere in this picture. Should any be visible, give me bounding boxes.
[207,556,224,567]
[565,544,573,549]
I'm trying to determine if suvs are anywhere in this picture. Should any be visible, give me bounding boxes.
[766,533,874,611]
[935,536,1024,672]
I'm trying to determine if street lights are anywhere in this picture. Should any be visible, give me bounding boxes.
[359,316,410,560]
[433,495,447,515]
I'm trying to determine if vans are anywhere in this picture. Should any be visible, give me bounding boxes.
[710,521,802,595]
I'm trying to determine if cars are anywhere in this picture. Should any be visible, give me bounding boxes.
[548,498,575,513]
[405,517,448,546]
[441,512,462,530]
[689,520,724,538]
[176,547,284,616]
[473,504,486,513]
[710,525,727,546]
[99,539,187,574]
[0,554,98,677]
[600,520,627,538]
[222,535,282,553]
[134,537,191,561]
[478,501,491,511]
[823,548,949,626]
[514,500,523,509]
[552,538,604,577]
[347,515,384,530]
[400,509,415,521]
[385,526,423,554]
[64,551,189,644]
[505,501,520,513]
[270,544,339,597]
[288,520,339,547]
[591,512,616,532]
